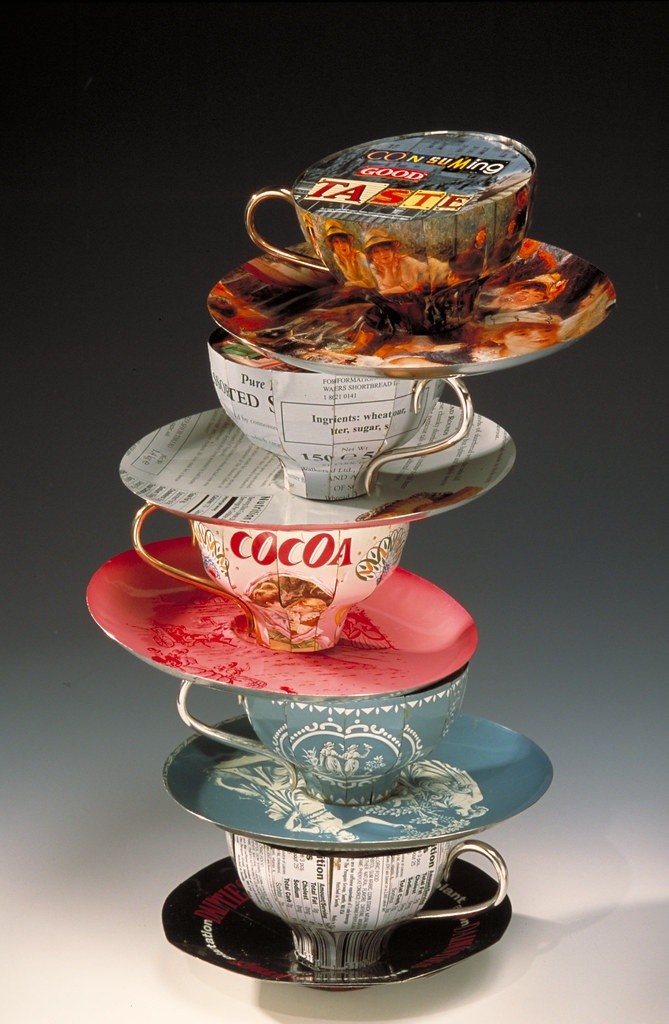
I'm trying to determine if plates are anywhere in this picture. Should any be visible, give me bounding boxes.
[116,401,520,534]
[157,716,558,850]
[85,536,479,696]
[160,855,513,990]
[204,237,617,384]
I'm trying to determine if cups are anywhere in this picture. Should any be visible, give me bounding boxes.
[174,666,469,806]
[225,831,507,969]
[125,503,411,656]
[206,343,473,500]
[244,134,537,334]
